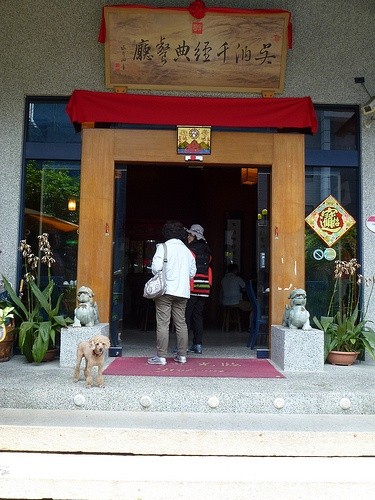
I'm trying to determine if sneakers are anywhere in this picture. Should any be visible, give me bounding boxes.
[188,344,203,354]
[147,356,167,365]
[174,355,187,363]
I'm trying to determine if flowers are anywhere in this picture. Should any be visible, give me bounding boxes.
[0,233,77,365]
[313,259,375,363]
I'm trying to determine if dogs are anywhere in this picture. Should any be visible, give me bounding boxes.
[73,334,113,390]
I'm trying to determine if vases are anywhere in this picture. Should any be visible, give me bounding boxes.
[23,334,35,363]
[326,351,360,366]
[41,347,58,363]
[0,326,15,362]
[62,299,76,311]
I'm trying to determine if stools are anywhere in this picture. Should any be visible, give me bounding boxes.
[140,300,156,331]
[221,305,241,337]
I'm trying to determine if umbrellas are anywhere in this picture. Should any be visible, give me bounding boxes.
[24,207,79,233]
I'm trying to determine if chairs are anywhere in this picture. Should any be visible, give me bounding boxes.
[247,280,268,349]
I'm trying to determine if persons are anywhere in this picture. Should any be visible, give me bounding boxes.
[172,224,213,355]
[147,221,197,366]
[220,264,253,312]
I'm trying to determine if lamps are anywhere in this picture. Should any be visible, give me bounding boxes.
[240,168,258,186]
[65,195,76,221]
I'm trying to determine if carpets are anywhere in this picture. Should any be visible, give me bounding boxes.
[102,357,286,378]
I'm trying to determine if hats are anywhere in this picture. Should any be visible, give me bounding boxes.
[183,224,207,244]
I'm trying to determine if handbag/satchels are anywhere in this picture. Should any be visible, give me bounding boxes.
[143,270,167,300]
